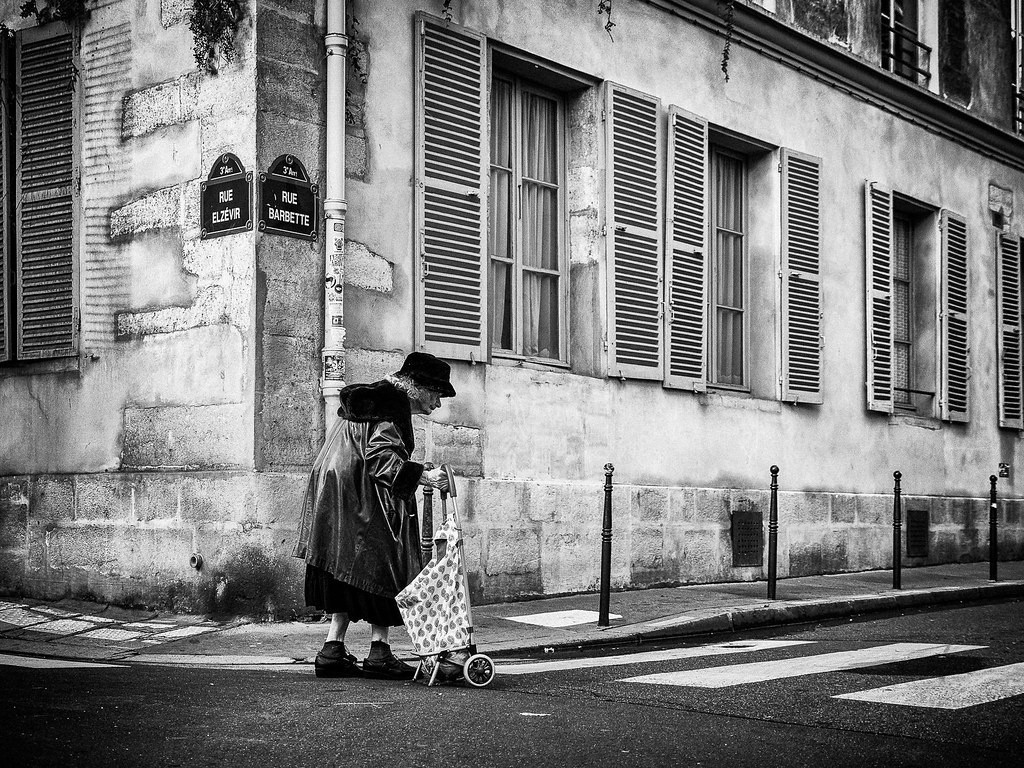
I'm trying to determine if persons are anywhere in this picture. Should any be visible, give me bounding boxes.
[292,351,456,682]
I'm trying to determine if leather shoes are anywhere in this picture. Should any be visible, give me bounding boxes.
[361,653,422,680]
[314,651,373,680]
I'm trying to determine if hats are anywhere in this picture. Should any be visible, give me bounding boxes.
[393,352,456,397]
